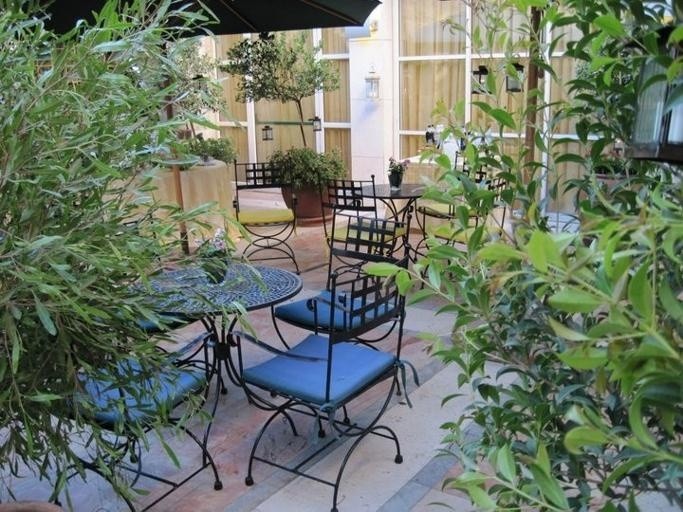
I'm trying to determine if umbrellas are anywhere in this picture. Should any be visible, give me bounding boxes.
[0,0,383,259]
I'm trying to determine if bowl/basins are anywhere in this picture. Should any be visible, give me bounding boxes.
[150,152,201,171]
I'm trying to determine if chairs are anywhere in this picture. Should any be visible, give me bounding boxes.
[229,156,299,278]
[316,151,511,288]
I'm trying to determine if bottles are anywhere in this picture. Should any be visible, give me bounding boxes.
[424,123,445,147]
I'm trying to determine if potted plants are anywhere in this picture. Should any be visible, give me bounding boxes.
[268,147,344,224]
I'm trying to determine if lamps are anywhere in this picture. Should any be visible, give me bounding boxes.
[260,125,273,141]
[619,18,683,167]
[470,64,523,95]
[362,65,380,100]
[311,116,321,132]
[344,20,377,40]
